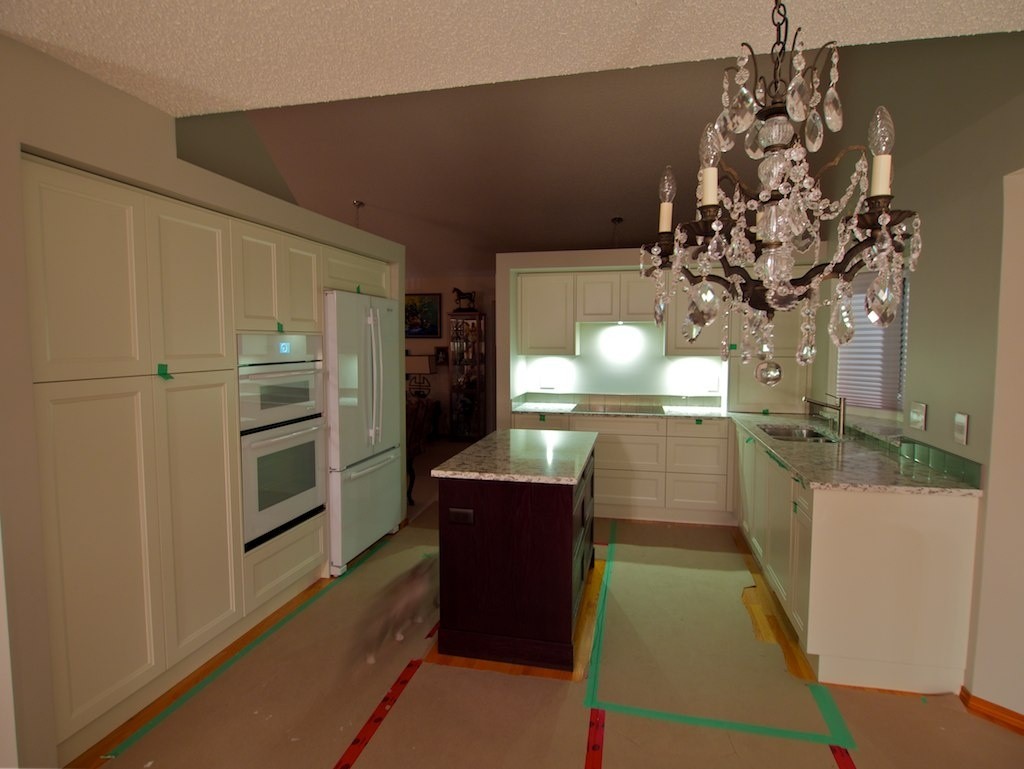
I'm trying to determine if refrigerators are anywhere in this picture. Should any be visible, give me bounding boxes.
[323,288,410,580]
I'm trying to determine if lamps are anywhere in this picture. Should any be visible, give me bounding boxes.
[638,0,926,388]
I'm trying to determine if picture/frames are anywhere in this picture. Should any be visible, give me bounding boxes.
[405,293,441,339]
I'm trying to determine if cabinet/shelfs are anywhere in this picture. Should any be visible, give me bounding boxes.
[428,426,598,673]
[230,214,326,337]
[576,269,662,324]
[515,414,981,698]
[17,144,238,385]
[446,311,488,442]
[669,267,812,417]
[243,510,331,617]
[517,272,581,357]
[27,367,239,745]
[324,244,391,297]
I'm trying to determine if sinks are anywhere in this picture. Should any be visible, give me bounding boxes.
[771,434,836,444]
[758,423,829,438]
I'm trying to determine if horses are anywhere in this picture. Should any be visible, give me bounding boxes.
[452,287,476,309]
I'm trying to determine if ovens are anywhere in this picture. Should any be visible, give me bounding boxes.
[237,329,325,548]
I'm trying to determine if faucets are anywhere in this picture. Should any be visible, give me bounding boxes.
[802,393,847,437]
[811,414,834,431]
